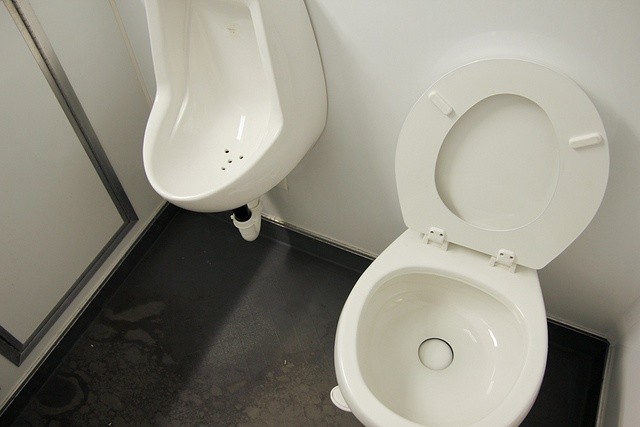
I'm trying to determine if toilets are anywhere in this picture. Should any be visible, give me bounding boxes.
[333,58,612,427]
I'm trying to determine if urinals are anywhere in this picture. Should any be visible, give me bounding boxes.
[141,1,329,243]
